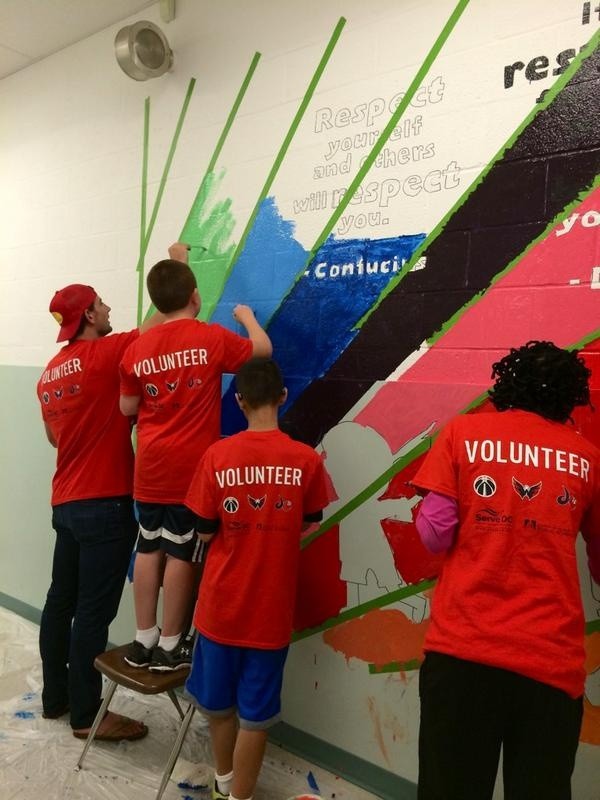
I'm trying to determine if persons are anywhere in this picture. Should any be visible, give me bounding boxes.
[173,357,338,800]
[409,340,599,800]
[34,237,191,739]
[109,260,276,676]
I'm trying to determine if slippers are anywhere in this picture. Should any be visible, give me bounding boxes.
[73,716,149,741]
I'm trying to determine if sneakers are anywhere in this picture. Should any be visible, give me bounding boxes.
[148,633,195,675]
[124,639,159,668]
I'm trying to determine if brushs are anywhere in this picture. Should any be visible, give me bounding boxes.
[187,244,207,253]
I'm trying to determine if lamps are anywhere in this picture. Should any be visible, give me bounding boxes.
[113,20,174,81]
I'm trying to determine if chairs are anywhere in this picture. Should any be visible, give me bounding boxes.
[76,553,241,800]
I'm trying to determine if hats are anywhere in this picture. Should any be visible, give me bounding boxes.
[48,283,98,343]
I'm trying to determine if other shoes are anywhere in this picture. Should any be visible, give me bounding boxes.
[212,779,230,800]
